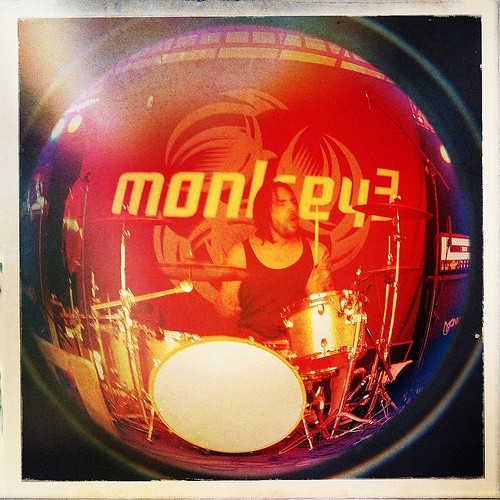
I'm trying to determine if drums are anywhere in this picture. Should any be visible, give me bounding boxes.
[281,290,367,372]
[149,336,305,453]
[107,322,144,396]
[145,329,198,360]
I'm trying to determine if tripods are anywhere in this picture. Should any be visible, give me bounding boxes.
[100,378,154,418]
[325,367,402,435]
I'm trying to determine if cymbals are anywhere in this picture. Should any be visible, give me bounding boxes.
[369,263,413,273]
[351,204,433,219]
[148,260,250,281]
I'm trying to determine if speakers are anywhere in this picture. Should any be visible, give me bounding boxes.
[409,274,471,391]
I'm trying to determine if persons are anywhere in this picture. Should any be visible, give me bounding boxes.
[223,181,357,426]
[45,175,90,335]
